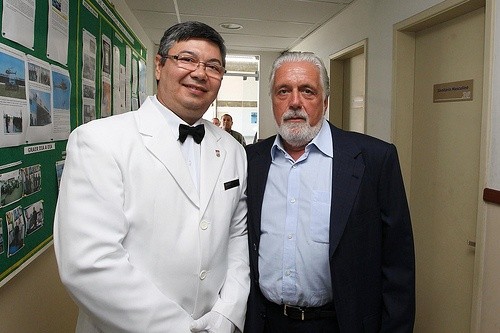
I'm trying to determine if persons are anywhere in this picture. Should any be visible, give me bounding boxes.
[5,114,23,133]
[55,21,252,333]
[242,51,417,332]
[212,117,221,127]
[1,164,44,248]
[220,114,248,148]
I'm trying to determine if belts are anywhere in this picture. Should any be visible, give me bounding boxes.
[257,294,334,320]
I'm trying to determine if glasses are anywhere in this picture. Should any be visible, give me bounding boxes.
[162,53,227,79]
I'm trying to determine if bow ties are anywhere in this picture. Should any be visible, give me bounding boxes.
[177,123,207,143]
